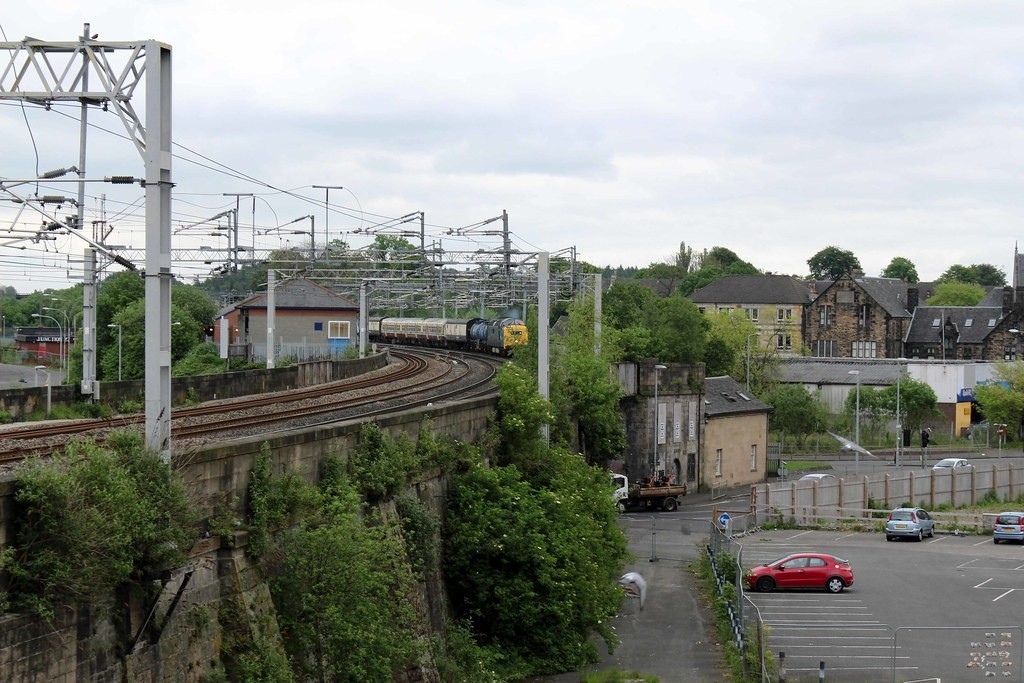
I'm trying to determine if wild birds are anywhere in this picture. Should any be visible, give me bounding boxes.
[826,430,877,459]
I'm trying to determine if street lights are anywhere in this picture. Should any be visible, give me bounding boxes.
[895,357,907,467]
[746,330,761,389]
[105,324,124,381]
[846,369,864,472]
[650,365,669,564]
[27,292,76,414]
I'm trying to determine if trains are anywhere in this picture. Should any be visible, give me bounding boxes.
[368,315,528,356]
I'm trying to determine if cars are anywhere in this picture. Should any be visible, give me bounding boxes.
[886,506,934,541]
[798,472,834,481]
[992,510,1024,545]
[747,552,854,594]
[932,458,970,469]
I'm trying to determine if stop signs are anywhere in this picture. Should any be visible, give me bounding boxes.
[998,425,1005,437]
